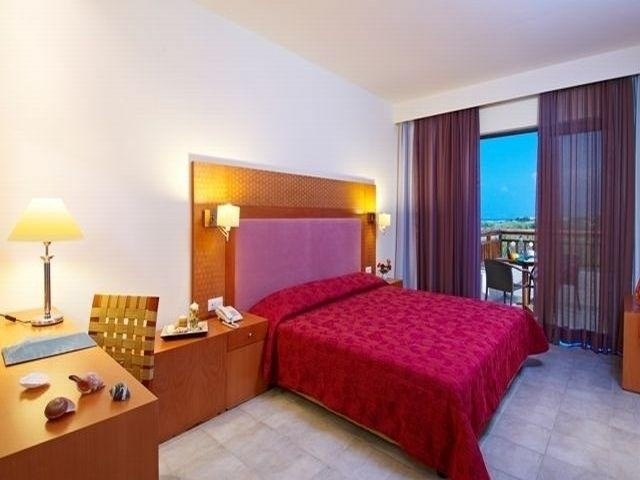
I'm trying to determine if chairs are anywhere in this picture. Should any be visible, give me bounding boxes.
[483,254,583,312]
[87,293,160,393]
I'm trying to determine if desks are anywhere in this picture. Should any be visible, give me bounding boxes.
[0,305,160,480]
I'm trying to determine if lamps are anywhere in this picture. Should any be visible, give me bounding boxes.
[367,211,390,231]
[202,203,241,242]
[8,196,83,327]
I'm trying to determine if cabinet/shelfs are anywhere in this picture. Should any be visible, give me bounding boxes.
[151,320,228,444]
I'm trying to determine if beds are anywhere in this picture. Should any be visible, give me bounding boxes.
[223,206,549,479]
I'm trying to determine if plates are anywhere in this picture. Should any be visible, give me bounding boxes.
[158,320,209,338]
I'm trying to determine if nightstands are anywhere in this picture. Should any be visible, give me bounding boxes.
[207,310,270,411]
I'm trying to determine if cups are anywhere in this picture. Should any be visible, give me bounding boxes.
[187,303,200,332]
[178,316,187,332]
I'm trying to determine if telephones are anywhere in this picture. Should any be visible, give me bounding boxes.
[215,305,243,324]
